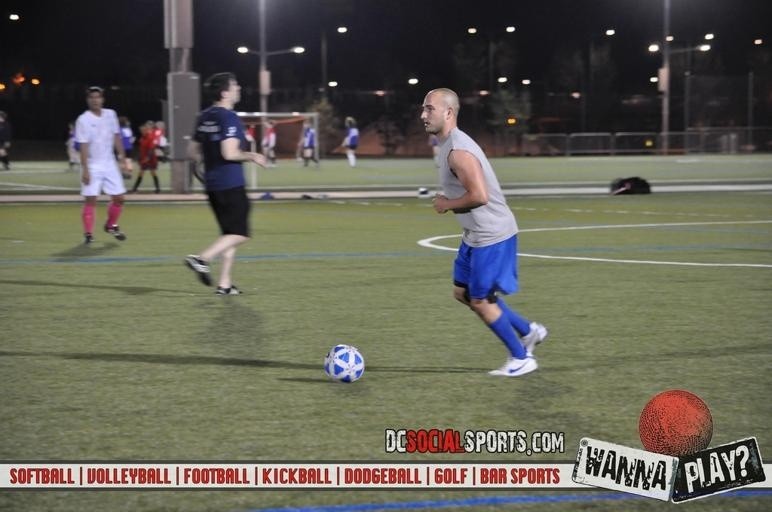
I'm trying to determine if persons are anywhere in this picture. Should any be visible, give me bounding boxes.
[341,116,360,168]
[0,110,12,169]
[302,121,318,166]
[421,88,548,378]
[75,86,131,243]
[262,123,277,160]
[184,73,268,296]
[66,116,167,192]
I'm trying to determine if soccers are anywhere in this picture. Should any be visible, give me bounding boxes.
[638,391,712,457]
[324,344,365,384]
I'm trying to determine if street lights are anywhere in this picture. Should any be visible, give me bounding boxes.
[602,26,616,113]
[667,32,716,152]
[746,37,761,144]
[648,43,712,156]
[1,11,21,22]
[319,22,349,99]
[466,26,518,89]
[236,43,308,110]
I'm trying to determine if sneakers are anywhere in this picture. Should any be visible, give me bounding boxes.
[215,285,242,295]
[104,223,125,240]
[183,254,211,285]
[520,321,548,353]
[488,356,537,377]
